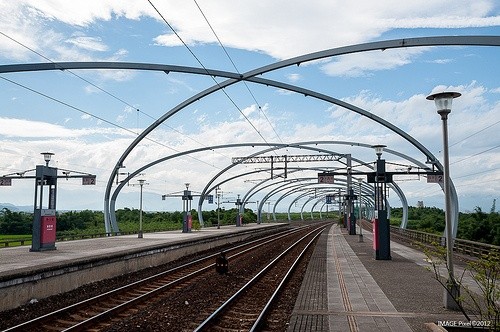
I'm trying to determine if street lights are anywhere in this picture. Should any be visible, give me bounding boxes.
[236,194,242,226]
[29,150,57,252]
[370,144,392,260]
[356,177,366,243]
[181,183,193,233]
[136,174,147,239]
[426,91,463,310]
